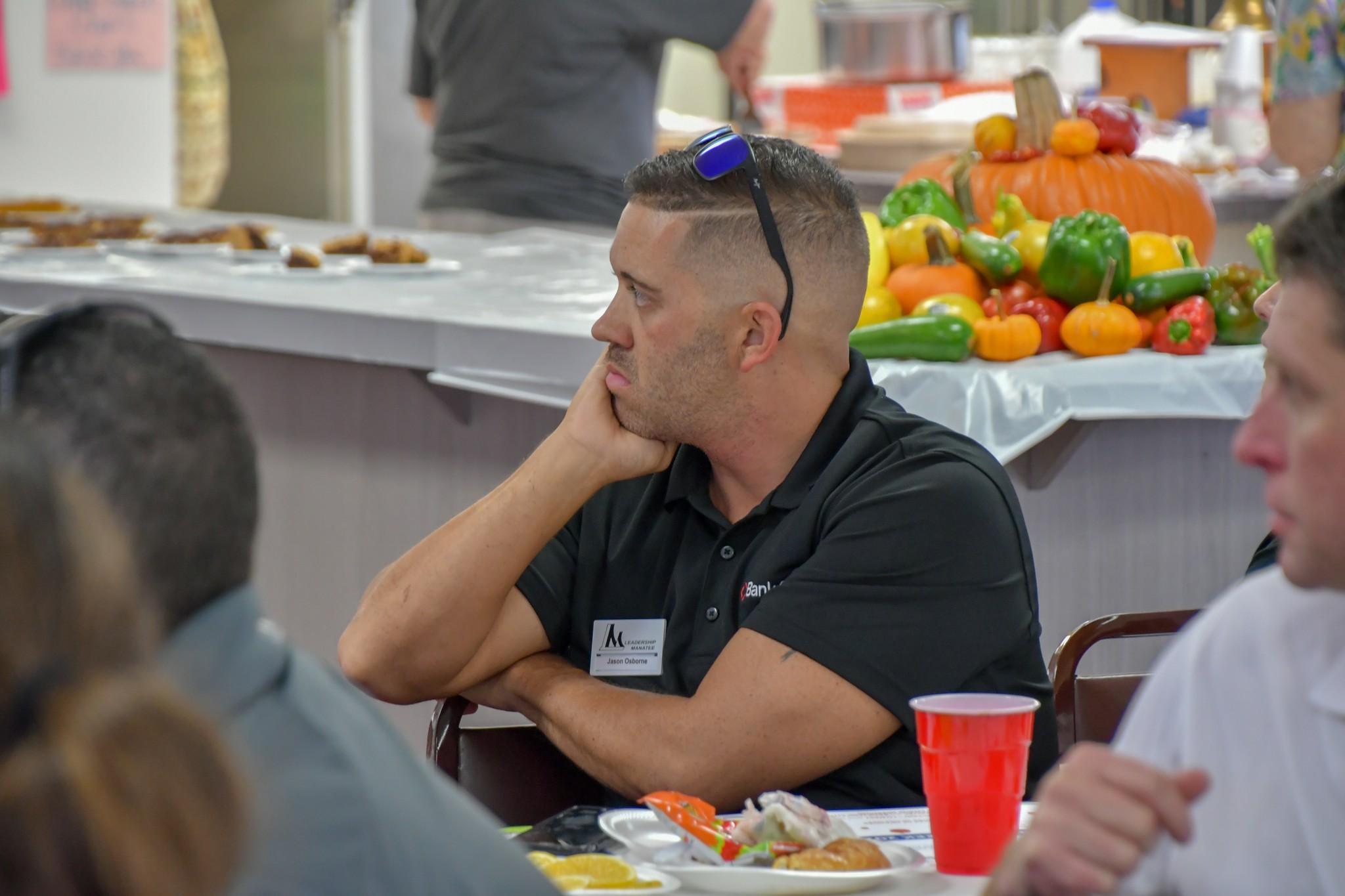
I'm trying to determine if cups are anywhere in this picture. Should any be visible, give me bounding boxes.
[908,691,1040,875]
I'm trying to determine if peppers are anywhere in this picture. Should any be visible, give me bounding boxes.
[982,277,1067,355]
[1123,221,1278,355]
[880,175,1130,305]
[846,281,985,364]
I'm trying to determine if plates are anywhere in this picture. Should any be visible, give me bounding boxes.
[551,856,682,896]
[594,806,925,896]
[1,207,460,280]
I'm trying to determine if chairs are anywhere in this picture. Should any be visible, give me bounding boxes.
[426,693,627,827]
[1046,604,1200,769]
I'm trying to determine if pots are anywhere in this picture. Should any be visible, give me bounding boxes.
[811,0,975,81]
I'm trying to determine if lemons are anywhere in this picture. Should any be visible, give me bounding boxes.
[527,850,663,892]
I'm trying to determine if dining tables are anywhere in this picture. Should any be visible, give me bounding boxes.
[500,799,1041,896]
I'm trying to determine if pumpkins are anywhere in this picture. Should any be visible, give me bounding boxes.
[883,223,983,313]
[1059,256,1143,358]
[975,98,1142,162]
[974,288,1042,361]
[897,66,1217,266]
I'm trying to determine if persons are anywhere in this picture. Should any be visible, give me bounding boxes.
[403,0,777,241]
[0,401,256,896]
[337,126,1056,812]
[979,173,1345,895]
[3,295,572,896]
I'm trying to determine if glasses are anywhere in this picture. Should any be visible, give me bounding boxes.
[0,299,168,416]
[685,122,793,343]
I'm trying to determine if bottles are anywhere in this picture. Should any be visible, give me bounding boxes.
[1056,0,1139,109]
